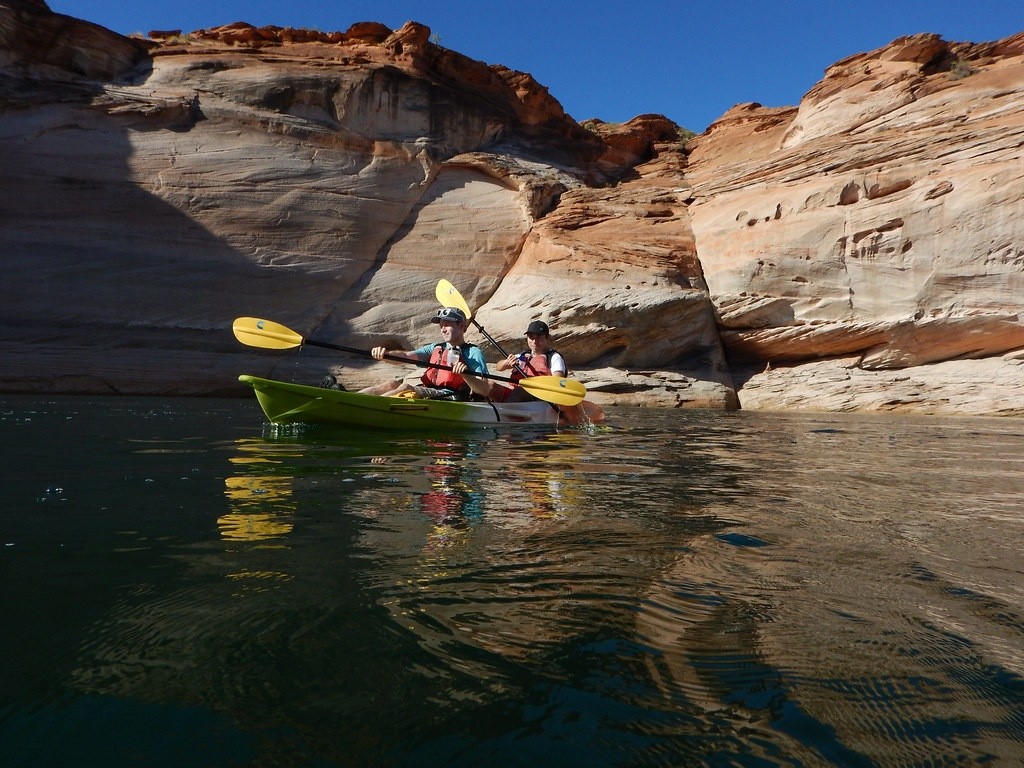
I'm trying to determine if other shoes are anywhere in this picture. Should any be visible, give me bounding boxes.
[319,376,336,388]
[331,383,347,391]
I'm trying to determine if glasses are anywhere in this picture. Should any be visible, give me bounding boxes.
[437,308,464,319]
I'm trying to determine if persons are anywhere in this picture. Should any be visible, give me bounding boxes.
[318,307,490,401]
[471,321,567,402]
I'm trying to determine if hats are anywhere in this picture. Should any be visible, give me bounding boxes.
[523,320,548,336]
[431,307,467,323]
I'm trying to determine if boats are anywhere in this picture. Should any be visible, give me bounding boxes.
[236,372,608,431]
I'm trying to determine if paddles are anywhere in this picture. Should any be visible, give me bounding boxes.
[434,277,568,420]
[231,315,588,408]
